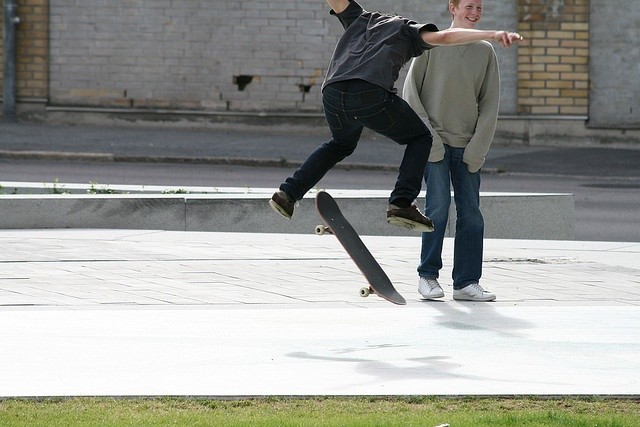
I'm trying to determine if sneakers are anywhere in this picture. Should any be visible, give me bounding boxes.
[386,200,435,232]
[453,283,496,301]
[268,191,299,220]
[418,277,444,299]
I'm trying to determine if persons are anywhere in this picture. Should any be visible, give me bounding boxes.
[402,0,501,302]
[268,0,524,232]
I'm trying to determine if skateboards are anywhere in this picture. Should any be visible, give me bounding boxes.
[315,191,406,305]
